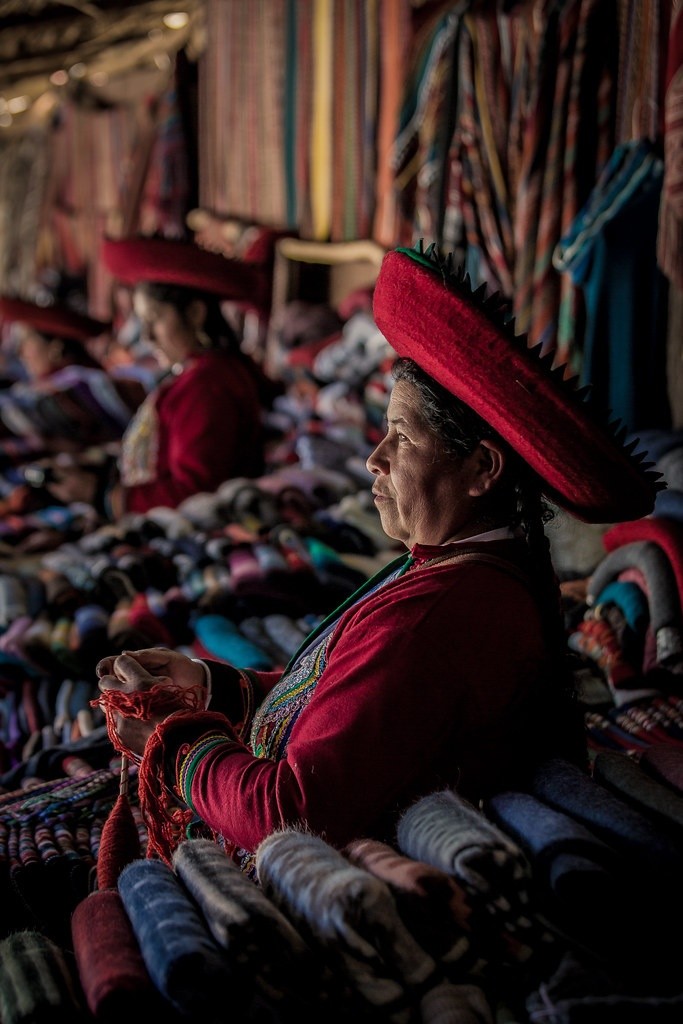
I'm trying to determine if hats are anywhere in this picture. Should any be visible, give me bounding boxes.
[373,238,669,524]
[99,228,268,305]
[0,296,107,341]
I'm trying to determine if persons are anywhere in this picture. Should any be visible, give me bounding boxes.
[93,247,683,847]
[0,218,264,524]
